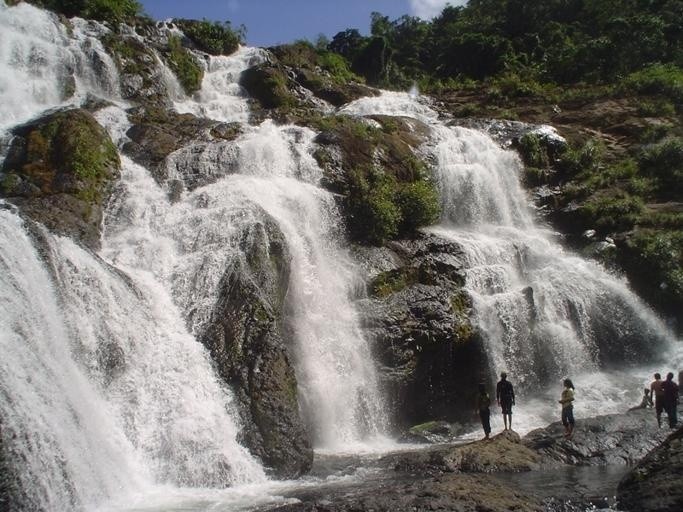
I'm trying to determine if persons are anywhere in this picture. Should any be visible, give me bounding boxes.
[473,384,491,440]
[648,373,667,430]
[558,378,575,437]
[626,388,654,412]
[495,371,515,431]
[659,372,678,428]
